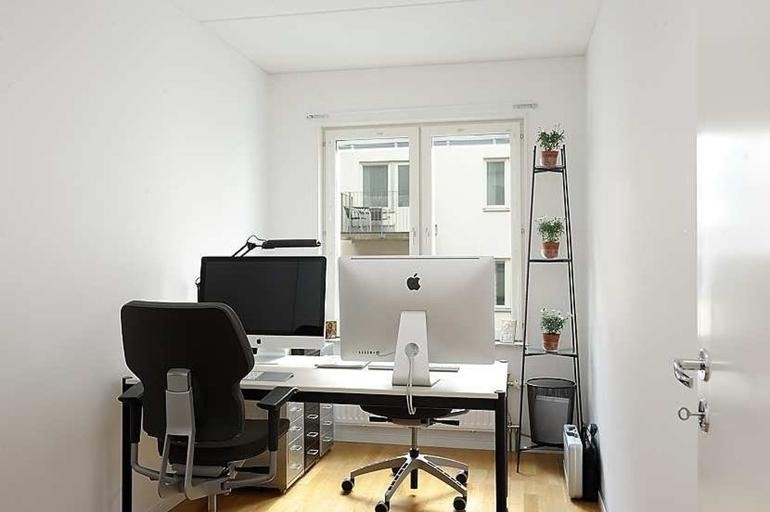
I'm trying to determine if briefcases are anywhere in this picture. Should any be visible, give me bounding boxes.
[563,424,584,498]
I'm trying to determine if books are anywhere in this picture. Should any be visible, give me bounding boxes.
[314,353,370,369]
[532,394,571,446]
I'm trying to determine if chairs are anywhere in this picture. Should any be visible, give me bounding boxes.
[341,403,470,512]
[344,205,394,231]
[118,302,300,512]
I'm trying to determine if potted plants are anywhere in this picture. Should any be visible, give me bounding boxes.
[535,122,566,166]
[539,308,572,352]
[535,215,566,258]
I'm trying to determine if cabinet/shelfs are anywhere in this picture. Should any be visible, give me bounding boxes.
[513,148,584,474]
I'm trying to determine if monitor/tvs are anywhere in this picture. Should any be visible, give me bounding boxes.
[337,254,496,388]
[196,255,328,364]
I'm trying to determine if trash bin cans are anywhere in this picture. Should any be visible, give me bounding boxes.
[526,377,577,446]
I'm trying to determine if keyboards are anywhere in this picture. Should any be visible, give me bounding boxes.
[243,370,294,382]
[366,361,458,372]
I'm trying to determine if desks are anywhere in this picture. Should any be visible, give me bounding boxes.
[122,357,509,512]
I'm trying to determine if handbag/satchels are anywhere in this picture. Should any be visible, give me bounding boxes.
[582,422,599,502]
